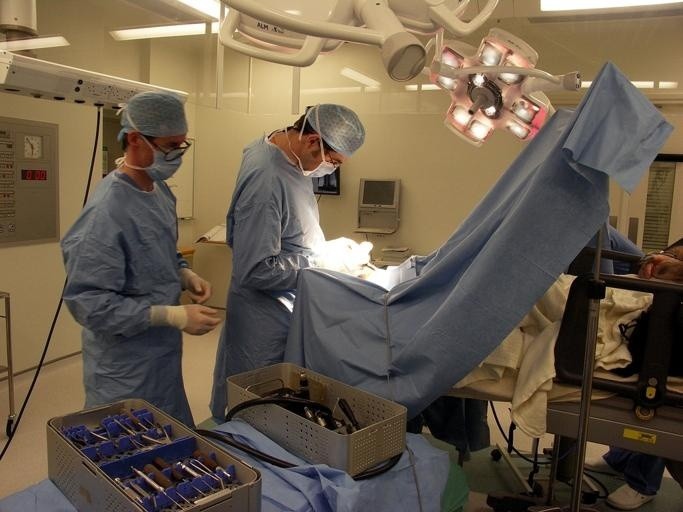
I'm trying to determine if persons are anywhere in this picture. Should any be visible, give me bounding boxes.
[582,237,683,509]
[585,226,665,275]
[60,90,223,431]
[209,104,365,420]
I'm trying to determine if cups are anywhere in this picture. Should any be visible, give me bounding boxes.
[151,140,190,161]
[328,153,342,168]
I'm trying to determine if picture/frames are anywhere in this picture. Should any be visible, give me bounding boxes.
[1,413,459,512]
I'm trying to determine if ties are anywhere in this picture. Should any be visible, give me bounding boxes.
[359,178,399,209]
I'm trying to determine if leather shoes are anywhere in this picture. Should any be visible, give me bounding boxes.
[315,236,373,277]
[151,305,221,336]
[180,268,211,304]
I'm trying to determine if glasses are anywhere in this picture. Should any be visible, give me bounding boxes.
[0,22,221,52]
[431,28,557,147]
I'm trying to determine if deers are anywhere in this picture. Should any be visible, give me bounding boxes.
[130,135,182,180]
[297,141,337,178]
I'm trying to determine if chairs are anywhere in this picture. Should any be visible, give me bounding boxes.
[583,460,655,510]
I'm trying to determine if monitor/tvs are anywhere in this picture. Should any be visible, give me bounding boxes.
[194,225,227,244]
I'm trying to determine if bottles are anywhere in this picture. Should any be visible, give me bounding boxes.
[120,92,188,137]
[308,104,365,158]
